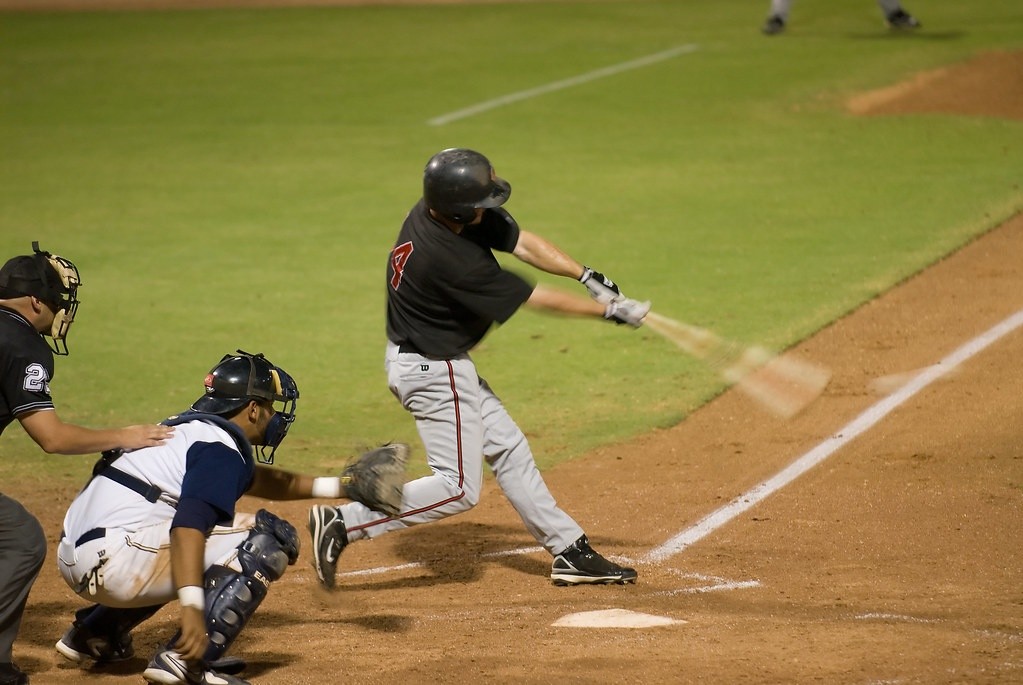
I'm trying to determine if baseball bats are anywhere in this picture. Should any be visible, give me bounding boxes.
[586,288,835,420]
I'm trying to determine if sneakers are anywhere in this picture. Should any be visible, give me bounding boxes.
[55,623,135,664]
[142,650,248,685]
[550,535,638,586]
[309,504,348,591]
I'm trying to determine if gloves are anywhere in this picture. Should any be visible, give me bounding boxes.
[577,267,619,305]
[602,297,653,329]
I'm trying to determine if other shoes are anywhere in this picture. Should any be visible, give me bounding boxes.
[885,10,922,31]
[0,661,27,685]
[762,17,784,36]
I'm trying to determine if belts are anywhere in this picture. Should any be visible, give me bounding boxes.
[398,343,426,353]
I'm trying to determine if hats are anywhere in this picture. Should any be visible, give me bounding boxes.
[0,256,63,306]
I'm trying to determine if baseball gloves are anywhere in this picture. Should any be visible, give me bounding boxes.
[337,437,411,520]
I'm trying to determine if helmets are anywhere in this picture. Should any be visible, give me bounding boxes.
[190,354,277,414]
[422,148,512,225]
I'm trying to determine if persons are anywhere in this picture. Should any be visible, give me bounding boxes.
[0,256,175,685]
[307,147,651,592]
[55,348,354,685]
[760,0,922,36]
